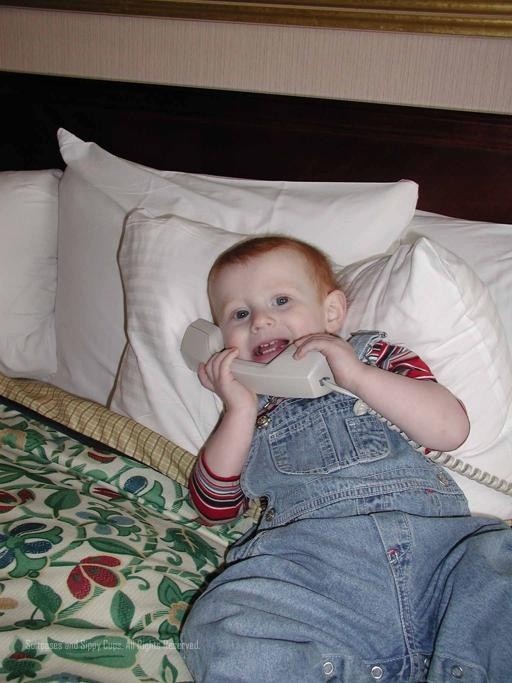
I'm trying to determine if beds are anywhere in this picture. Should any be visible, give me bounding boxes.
[0,68,512,683]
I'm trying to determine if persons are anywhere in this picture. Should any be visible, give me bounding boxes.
[181,236,511,683]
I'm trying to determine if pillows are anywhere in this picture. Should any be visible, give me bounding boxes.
[107,209,512,465]
[0,170,63,383]
[56,126,419,405]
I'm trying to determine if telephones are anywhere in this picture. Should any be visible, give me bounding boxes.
[181,320,512,496]
